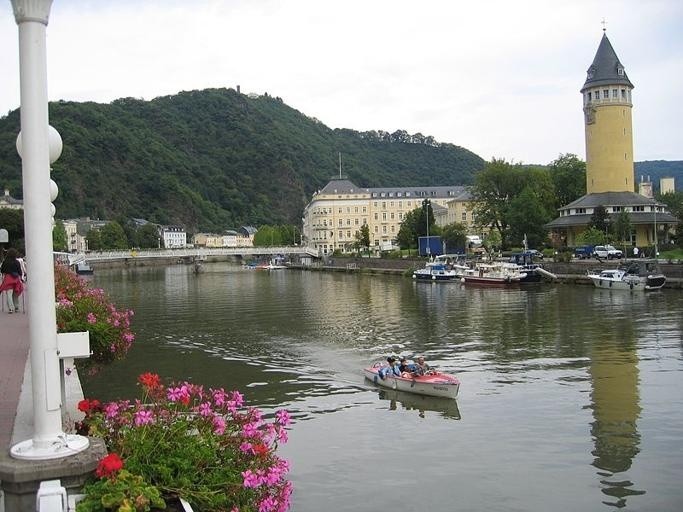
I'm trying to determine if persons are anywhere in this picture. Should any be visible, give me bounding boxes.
[639,250,645,257]
[0,247,24,314]
[378,356,435,382]
[632,244,638,257]
[15,254,26,283]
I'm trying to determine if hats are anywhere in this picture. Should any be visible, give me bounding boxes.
[387,357,393,362]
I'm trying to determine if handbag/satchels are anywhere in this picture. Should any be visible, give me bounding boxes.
[12,271,19,277]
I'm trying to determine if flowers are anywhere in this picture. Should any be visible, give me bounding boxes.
[55,264,136,369]
[72,370,294,512]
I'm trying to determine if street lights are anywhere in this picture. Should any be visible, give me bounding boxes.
[425,202,430,258]
[11,120,65,238]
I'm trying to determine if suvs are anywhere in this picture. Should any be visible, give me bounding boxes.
[557,242,623,260]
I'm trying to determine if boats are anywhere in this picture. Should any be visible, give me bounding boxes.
[413,234,543,287]
[75,264,95,274]
[364,363,461,399]
[584,268,667,290]
[192,258,205,275]
